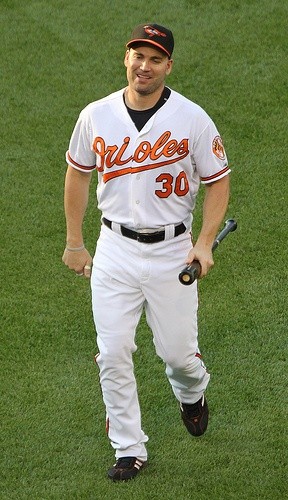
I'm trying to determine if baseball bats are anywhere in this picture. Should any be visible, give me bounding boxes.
[178,217,239,286]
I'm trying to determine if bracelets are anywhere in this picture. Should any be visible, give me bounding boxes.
[66,244,85,251]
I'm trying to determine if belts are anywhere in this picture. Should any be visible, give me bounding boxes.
[102,217,186,243]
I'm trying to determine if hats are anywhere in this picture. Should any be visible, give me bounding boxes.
[126,24,174,60]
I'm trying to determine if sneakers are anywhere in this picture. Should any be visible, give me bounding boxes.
[180,394,209,437]
[108,457,143,481]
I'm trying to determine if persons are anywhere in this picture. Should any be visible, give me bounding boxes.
[62,24,237,482]
